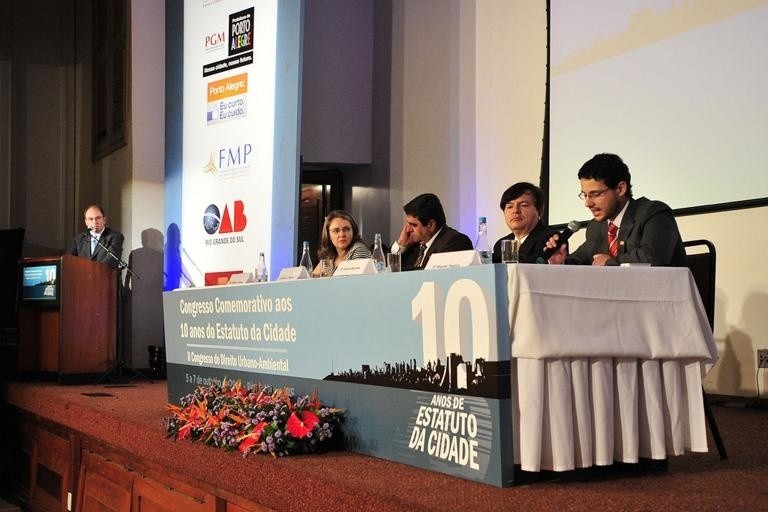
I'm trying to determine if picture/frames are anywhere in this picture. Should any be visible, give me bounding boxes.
[90,0,131,164]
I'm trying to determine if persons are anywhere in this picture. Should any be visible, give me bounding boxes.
[61,201,124,267]
[309,209,373,278]
[543,152,688,267]
[383,193,473,271]
[477,181,568,264]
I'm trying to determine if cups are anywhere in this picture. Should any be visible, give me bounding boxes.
[320,260,333,277]
[386,253,401,272]
[500,239,519,263]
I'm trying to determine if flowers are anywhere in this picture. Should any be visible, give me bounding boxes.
[164,376,347,458]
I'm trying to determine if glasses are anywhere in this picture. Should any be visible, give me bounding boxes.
[578,188,610,201]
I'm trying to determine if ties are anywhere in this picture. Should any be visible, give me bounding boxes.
[608,221,619,257]
[92,235,98,252]
[413,244,427,267]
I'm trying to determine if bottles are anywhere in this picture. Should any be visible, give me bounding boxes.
[255,252,268,282]
[300,241,313,278]
[475,217,493,264]
[372,234,386,273]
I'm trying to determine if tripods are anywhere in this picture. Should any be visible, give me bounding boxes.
[92,236,154,384]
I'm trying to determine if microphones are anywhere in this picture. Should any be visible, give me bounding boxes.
[536,220,580,263]
[80,226,93,239]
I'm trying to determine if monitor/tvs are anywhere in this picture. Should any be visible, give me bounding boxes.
[19,260,59,308]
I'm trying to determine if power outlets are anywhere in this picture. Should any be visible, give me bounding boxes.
[758,349,768,368]
